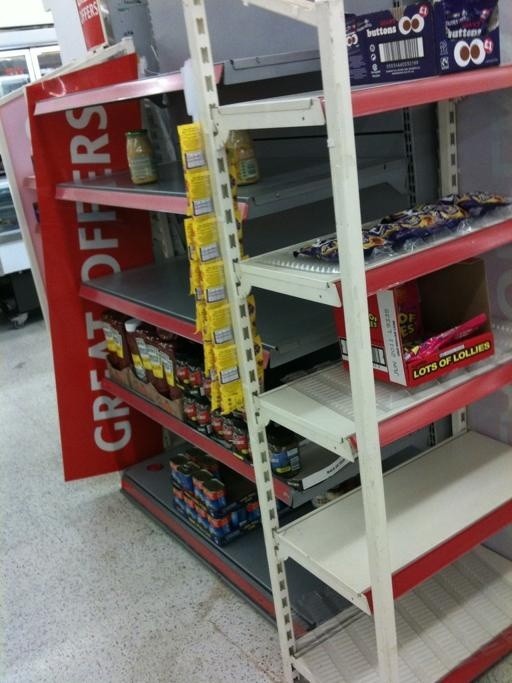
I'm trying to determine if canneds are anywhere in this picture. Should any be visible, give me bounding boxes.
[169,447,291,547]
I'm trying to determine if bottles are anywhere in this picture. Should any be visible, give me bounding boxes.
[223,417,234,443]
[183,397,196,422]
[234,422,251,457]
[211,410,225,438]
[197,399,210,426]
[173,355,189,389]
[266,425,301,478]
[189,366,202,388]
